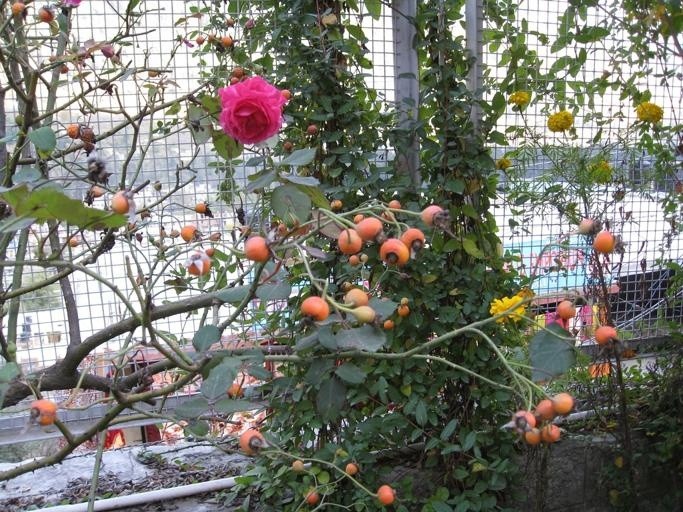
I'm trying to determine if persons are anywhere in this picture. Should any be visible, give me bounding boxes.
[22,316,33,348]
